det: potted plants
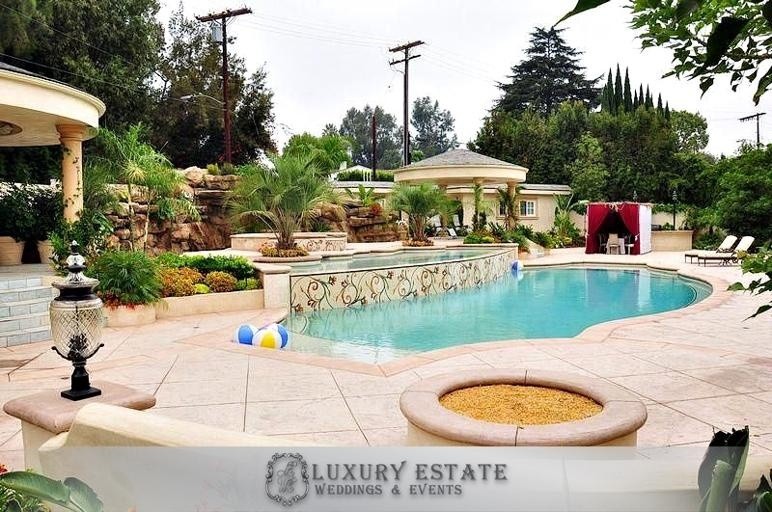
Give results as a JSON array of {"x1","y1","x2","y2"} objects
[{"x1": 0, "y1": 172, "x2": 71, "y2": 267}]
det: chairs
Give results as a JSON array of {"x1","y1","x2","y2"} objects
[
  {"x1": 684, "y1": 234, "x2": 755, "y2": 267},
  {"x1": 598, "y1": 232, "x2": 634, "y2": 255},
  {"x1": 431, "y1": 215, "x2": 469, "y2": 240}
]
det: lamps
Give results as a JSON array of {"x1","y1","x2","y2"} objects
[{"x1": 49, "y1": 238, "x2": 107, "y2": 401}]
